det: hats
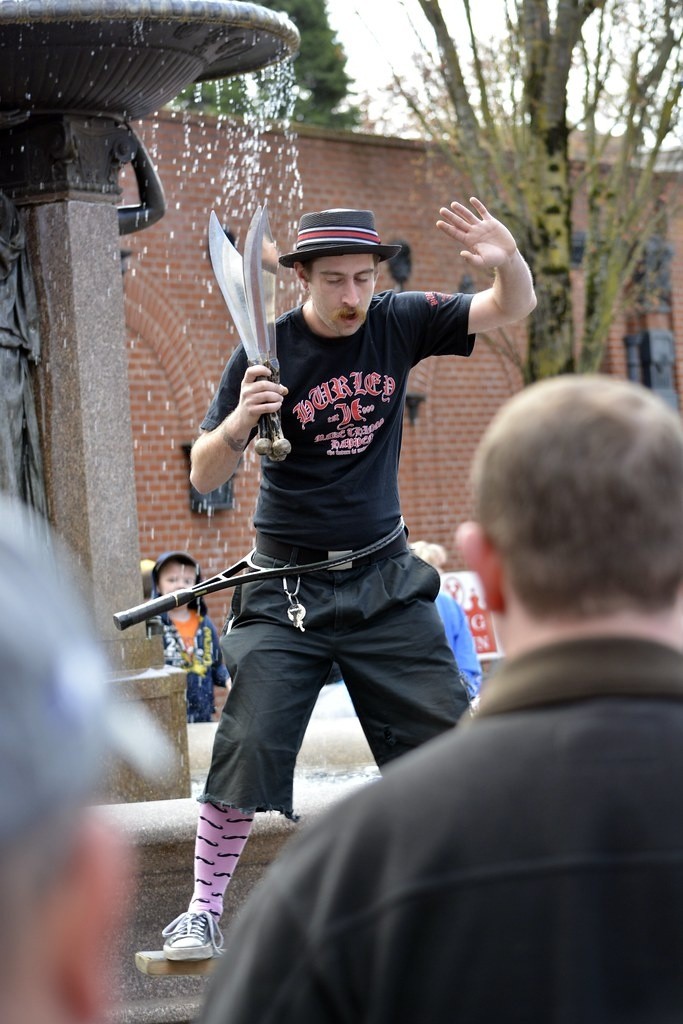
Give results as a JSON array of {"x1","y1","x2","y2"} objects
[{"x1": 278, "y1": 209, "x2": 403, "y2": 268}]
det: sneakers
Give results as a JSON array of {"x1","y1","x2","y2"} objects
[{"x1": 163, "y1": 911, "x2": 224, "y2": 961}]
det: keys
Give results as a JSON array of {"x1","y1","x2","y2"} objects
[{"x1": 285, "y1": 604, "x2": 306, "y2": 632}]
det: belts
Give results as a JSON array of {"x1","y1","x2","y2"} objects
[{"x1": 255, "y1": 529, "x2": 408, "y2": 569}]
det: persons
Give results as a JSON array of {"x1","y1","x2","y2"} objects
[
  {"x1": 194, "y1": 372, "x2": 682, "y2": 1024},
  {"x1": 146, "y1": 551, "x2": 232, "y2": 723},
  {"x1": 164, "y1": 195, "x2": 537, "y2": 962},
  {"x1": 0, "y1": 500, "x2": 141, "y2": 1024},
  {"x1": 409, "y1": 541, "x2": 481, "y2": 700}
]
